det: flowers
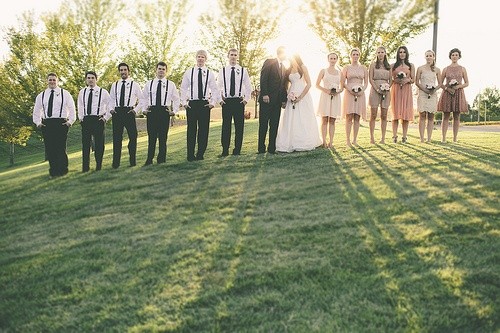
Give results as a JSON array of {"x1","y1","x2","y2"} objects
[
  {"x1": 426, "y1": 85, "x2": 435, "y2": 99},
  {"x1": 448, "y1": 79, "x2": 457, "y2": 96},
  {"x1": 287, "y1": 90, "x2": 297, "y2": 109},
  {"x1": 331, "y1": 87, "x2": 336, "y2": 100},
  {"x1": 396, "y1": 71, "x2": 407, "y2": 87},
  {"x1": 380, "y1": 83, "x2": 390, "y2": 99},
  {"x1": 352, "y1": 86, "x2": 362, "y2": 102}
]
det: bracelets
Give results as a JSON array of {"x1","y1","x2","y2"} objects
[
  {"x1": 377, "y1": 89, "x2": 379, "y2": 91},
  {"x1": 445, "y1": 87, "x2": 448, "y2": 91}
]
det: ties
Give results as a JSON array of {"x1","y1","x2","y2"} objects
[
  {"x1": 156, "y1": 80, "x2": 162, "y2": 112},
  {"x1": 230, "y1": 67, "x2": 236, "y2": 96},
  {"x1": 87, "y1": 89, "x2": 94, "y2": 116},
  {"x1": 120, "y1": 80, "x2": 126, "y2": 107},
  {"x1": 198, "y1": 67, "x2": 203, "y2": 100},
  {"x1": 279, "y1": 62, "x2": 283, "y2": 79},
  {"x1": 47, "y1": 90, "x2": 55, "y2": 117}
]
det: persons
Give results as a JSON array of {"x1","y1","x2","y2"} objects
[
  {"x1": 32, "y1": 73, "x2": 76, "y2": 180},
  {"x1": 341, "y1": 49, "x2": 368, "y2": 147},
  {"x1": 416, "y1": 50, "x2": 441, "y2": 144},
  {"x1": 218, "y1": 49, "x2": 250, "y2": 158},
  {"x1": 255, "y1": 46, "x2": 288, "y2": 154},
  {"x1": 181, "y1": 50, "x2": 217, "y2": 161},
  {"x1": 275, "y1": 51, "x2": 321, "y2": 153},
  {"x1": 142, "y1": 62, "x2": 180, "y2": 165},
  {"x1": 77, "y1": 71, "x2": 110, "y2": 172},
  {"x1": 437, "y1": 48, "x2": 469, "y2": 144},
  {"x1": 368, "y1": 47, "x2": 391, "y2": 145},
  {"x1": 390, "y1": 46, "x2": 415, "y2": 143},
  {"x1": 315, "y1": 53, "x2": 341, "y2": 148},
  {"x1": 109, "y1": 63, "x2": 143, "y2": 168}
]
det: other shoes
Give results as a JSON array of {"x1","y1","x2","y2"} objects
[
  {"x1": 323, "y1": 143, "x2": 332, "y2": 148},
  {"x1": 441, "y1": 138, "x2": 447, "y2": 144},
  {"x1": 392, "y1": 135, "x2": 407, "y2": 144},
  {"x1": 423, "y1": 138, "x2": 432, "y2": 144}
]
